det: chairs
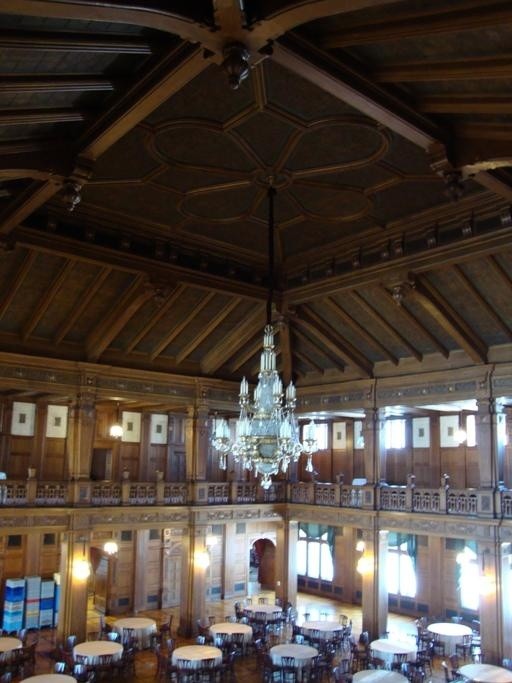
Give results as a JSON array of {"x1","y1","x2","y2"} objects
[
  {"x1": 155, "y1": 612, "x2": 512, "y2": 683},
  {"x1": 99, "y1": 615, "x2": 173, "y2": 653},
  {"x1": 0, "y1": 627, "x2": 138, "y2": 683},
  {"x1": 234, "y1": 597, "x2": 292, "y2": 631}
]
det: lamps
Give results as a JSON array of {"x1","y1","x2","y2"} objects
[
  {"x1": 59, "y1": 175, "x2": 86, "y2": 212},
  {"x1": 219, "y1": 37, "x2": 254, "y2": 92},
  {"x1": 208, "y1": 181, "x2": 318, "y2": 490}
]
